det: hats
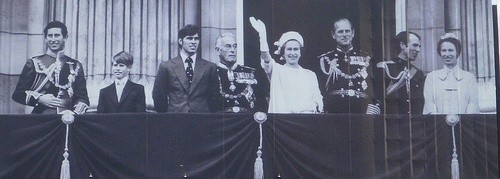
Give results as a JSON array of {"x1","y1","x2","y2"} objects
[
  {"x1": 441, "y1": 33, "x2": 458, "y2": 39},
  {"x1": 274, "y1": 31, "x2": 304, "y2": 54}
]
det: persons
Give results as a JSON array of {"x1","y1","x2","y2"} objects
[
  {"x1": 209, "y1": 32, "x2": 268, "y2": 114},
  {"x1": 152, "y1": 24, "x2": 220, "y2": 113},
  {"x1": 377, "y1": 31, "x2": 425, "y2": 114},
  {"x1": 12, "y1": 20, "x2": 90, "y2": 115},
  {"x1": 97, "y1": 51, "x2": 145, "y2": 113},
  {"x1": 422, "y1": 37, "x2": 481, "y2": 114},
  {"x1": 249, "y1": 16, "x2": 324, "y2": 114},
  {"x1": 314, "y1": 17, "x2": 382, "y2": 114}
]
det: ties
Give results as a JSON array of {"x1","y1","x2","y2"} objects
[
  {"x1": 117, "y1": 81, "x2": 124, "y2": 103},
  {"x1": 185, "y1": 57, "x2": 193, "y2": 89}
]
